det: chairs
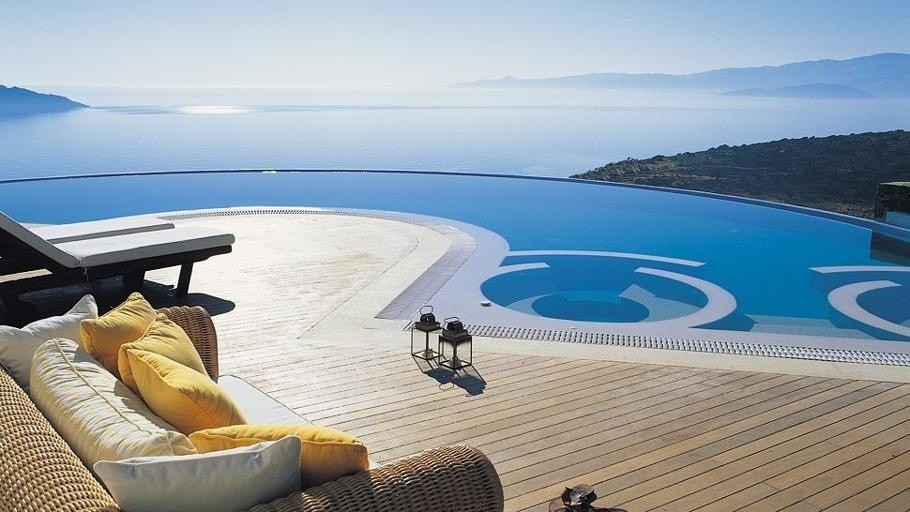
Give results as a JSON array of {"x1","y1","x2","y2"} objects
[
  {"x1": 28, "y1": 214, "x2": 176, "y2": 241},
  {"x1": 1, "y1": 210, "x2": 235, "y2": 306}
]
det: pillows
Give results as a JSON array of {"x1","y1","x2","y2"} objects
[
  {"x1": 118, "y1": 312, "x2": 209, "y2": 399},
  {"x1": 1, "y1": 292, "x2": 96, "y2": 389},
  {"x1": 22, "y1": 334, "x2": 197, "y2": 471},
  {"x1": 81, "y1": 291, "x2": 158, "y2": 378},
  {"x1": 191, "y1": 421, "x2": 369, "y2": 489},
  {"x1": 124, "y1": 347, "x2": 245, "y2": 438},
  {"x1": 91, "y1": 434, "x2": 303, "y2": 512}
]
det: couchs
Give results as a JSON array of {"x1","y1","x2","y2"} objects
[{"x1": 0, "y1": 292, "x2": 505, "y2": 511}]
